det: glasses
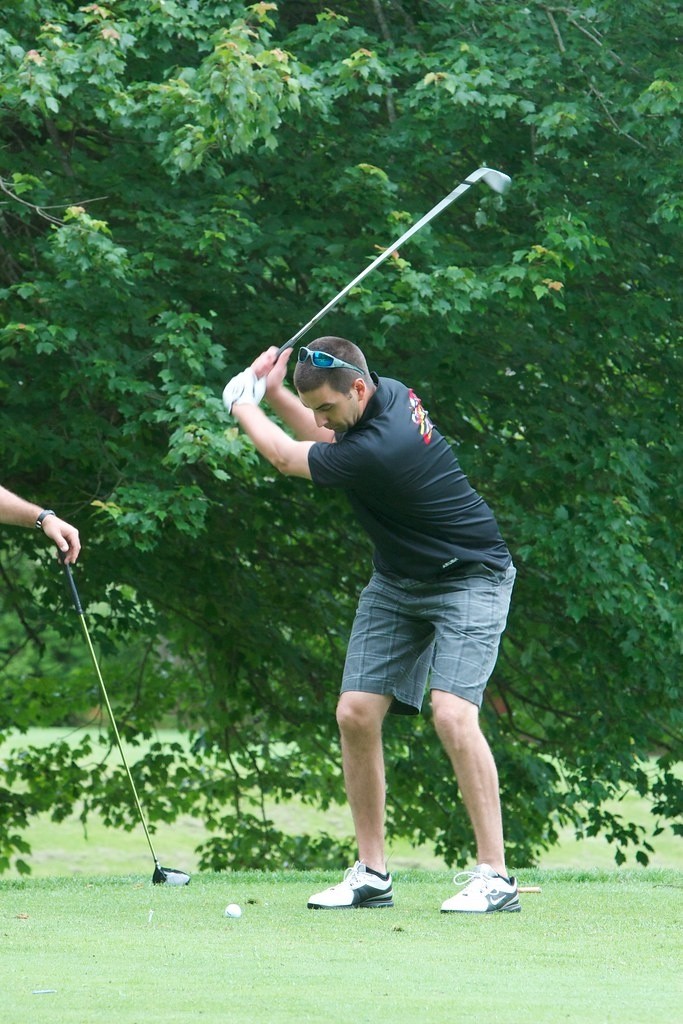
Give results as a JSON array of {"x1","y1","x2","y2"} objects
[{"x1": 298, "y1": 347, "x2": 365, "y2": 376}]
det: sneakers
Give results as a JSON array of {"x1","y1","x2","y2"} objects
[
  {"x1": 307, "y1": 858, "x2": 394, "y2": 909},
  {"x1": 441, "y1": 864, "x2": 521, "y2": 914}
]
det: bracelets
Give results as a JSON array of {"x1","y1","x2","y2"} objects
[{"x1": 35, "y1": 509, "x2": 55, "y2": 532}]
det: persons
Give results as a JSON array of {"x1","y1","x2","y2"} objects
[
  {"x1": 0, "y1": 486, "x2": 81, "y2": 564},
  {"x1": 223, "y1": 336, "x2": 521, "y2": 916}
]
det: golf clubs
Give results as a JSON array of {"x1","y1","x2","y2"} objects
[
  {"x1": 62, "y1": 551, "x2": 189, "y2": 885},
  {"x1": 274, "y1": 167, "x2": 511, "y2": 358}
]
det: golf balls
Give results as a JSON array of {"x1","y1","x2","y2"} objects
[{"x1": 225, "y1": 904, "x2": 242, "y2": 918}]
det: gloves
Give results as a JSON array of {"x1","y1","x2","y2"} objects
[{"x1": 222, "y1": 367, "x2": 265, "y2": 415}]
